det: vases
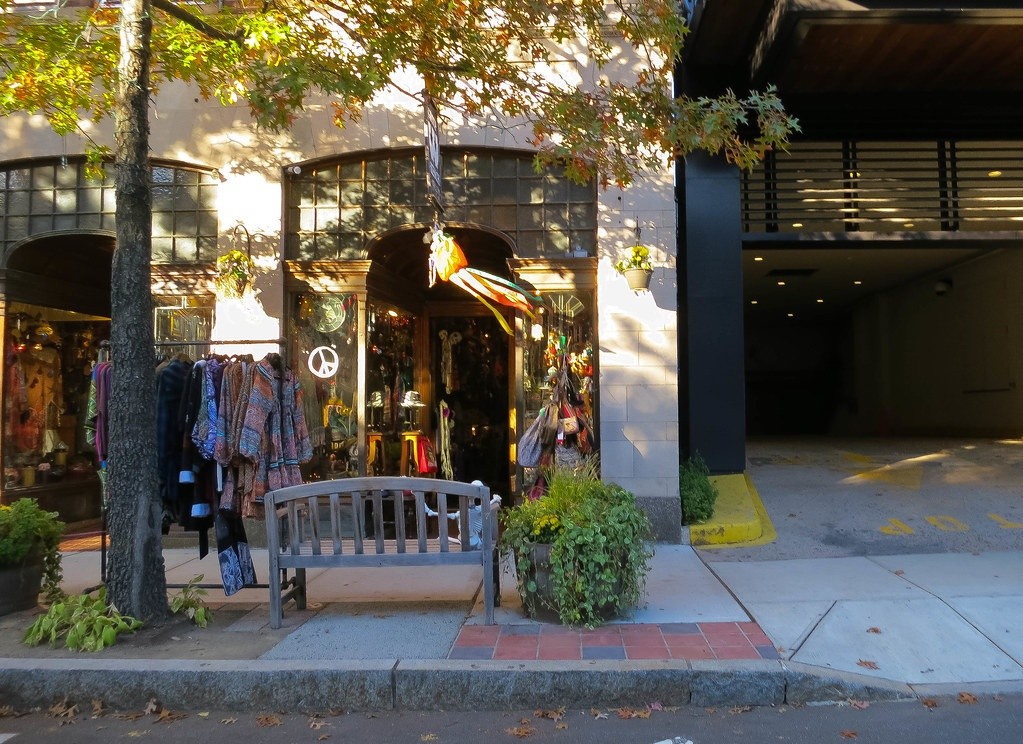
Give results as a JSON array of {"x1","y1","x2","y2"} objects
[
  {"x1": 513, "y1": 541, "x2": 625, "y2": 626},
  {"x1": 0, "y1": 555, "x2": 43, "y2": 615}
]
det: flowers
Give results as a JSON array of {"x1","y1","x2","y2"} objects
[
  {"x1": 0, "y1": 496, "x2": 66, "y2": 605},
  {"x1": 493, "y1": 450, "x2": 659, "y2": 632}
]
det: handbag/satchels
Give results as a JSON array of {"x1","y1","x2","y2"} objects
[
  {"x1": 416, "y1": 434, "x2": 439, "y2": 476},
  {"x1": 517, "y1": 365, "x2": 594, "y2": 468}
]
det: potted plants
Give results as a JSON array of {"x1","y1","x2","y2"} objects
[
  {"x1": 615, "y1": 244, "x2": 655, "y2": 292},
  {"x1": 214, "y1": 249, "x2": 253, "y2": 298}
]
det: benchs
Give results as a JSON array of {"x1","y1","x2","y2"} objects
[{"x1": 265, "y1": 475, "x2": 502, "y2": 629}]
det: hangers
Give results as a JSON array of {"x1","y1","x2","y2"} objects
[{"x1": 155, "y1": 341, "x2": 289, "y2": 371}]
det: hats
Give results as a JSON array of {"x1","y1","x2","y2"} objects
[
  {"x1": 367, "y1": 391, "x2": 384, "y2": 408},
  {"x1": 400, "y1": 390, "x2": 427, "y2": 408}
]
